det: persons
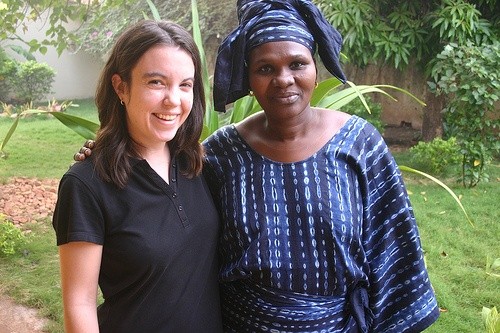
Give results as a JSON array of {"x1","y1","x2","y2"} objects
[
  {"x1": 72, "y1": 0, "x2": 440, "y2": 333},
  {"x1": 52, "y1": 18, "x2": 226, "y2": 333}
]
